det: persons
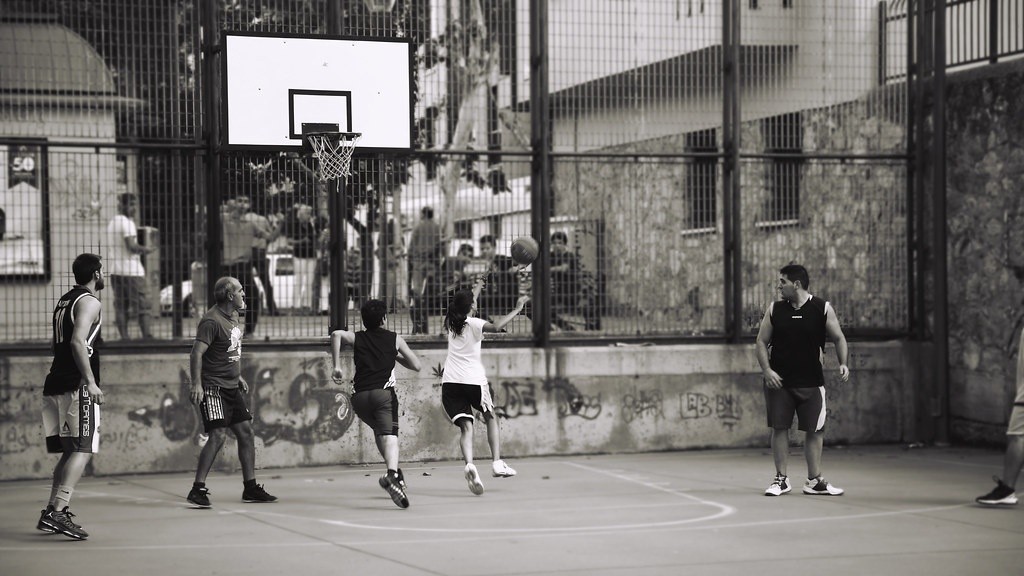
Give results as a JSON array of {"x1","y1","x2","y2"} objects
[
  {"x1": 976, "y1": 327, "x2": 1024, "y2": 505},
  {"x1": 35, "y1": 193, "x2": 580, "y2": 541},
  {"x1": 755, "y1": 264, "x2": 849, "y2": 497}
]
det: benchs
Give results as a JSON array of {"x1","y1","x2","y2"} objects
[{"x1": 423, "y1": 271, "x2": 593, "y2": 333}]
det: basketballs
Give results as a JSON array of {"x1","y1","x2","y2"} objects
[{"x1": 510, "y1": 236, "x2": 540, "y2": 264}]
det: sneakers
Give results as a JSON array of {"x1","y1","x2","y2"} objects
[
  {"x1": 464, "y1": 463, "x2": 484, "y2": 495},
  {"x1": 379, "y1": 470, "x2": 408, "y2": 508},
  {"x1": 802, "y1": 472, "x2": 843, "y2": 495},
  {"x1": 242, "y1": 484, "x2": 279, "y2": 503},
  {"x1": 41, "y1": 505, "x2": 89, "y2": 539},
  {"x1": 37, "y1": 510, "x2": 63, "y2": 533},
  {"x1": 492, "y1": 460, "x2": 517, "y2": 477},
  {"x1": 976, "y1": 476, "x2": 1018, "y2": 504},
  {"x1": 764, "y1": 471, "x2": 792, "y2": 495},
  {"x1": 186, "y1": 486, "x2": 212, "y2": 507}
]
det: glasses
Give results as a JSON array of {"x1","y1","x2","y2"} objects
[{"x1": 462, "y1": 251, "x2": 472, "y2": 258}]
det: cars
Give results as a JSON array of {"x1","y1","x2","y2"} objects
[{"x1": 156, "y1": 252, "x2": 355, "y2": 320}]
